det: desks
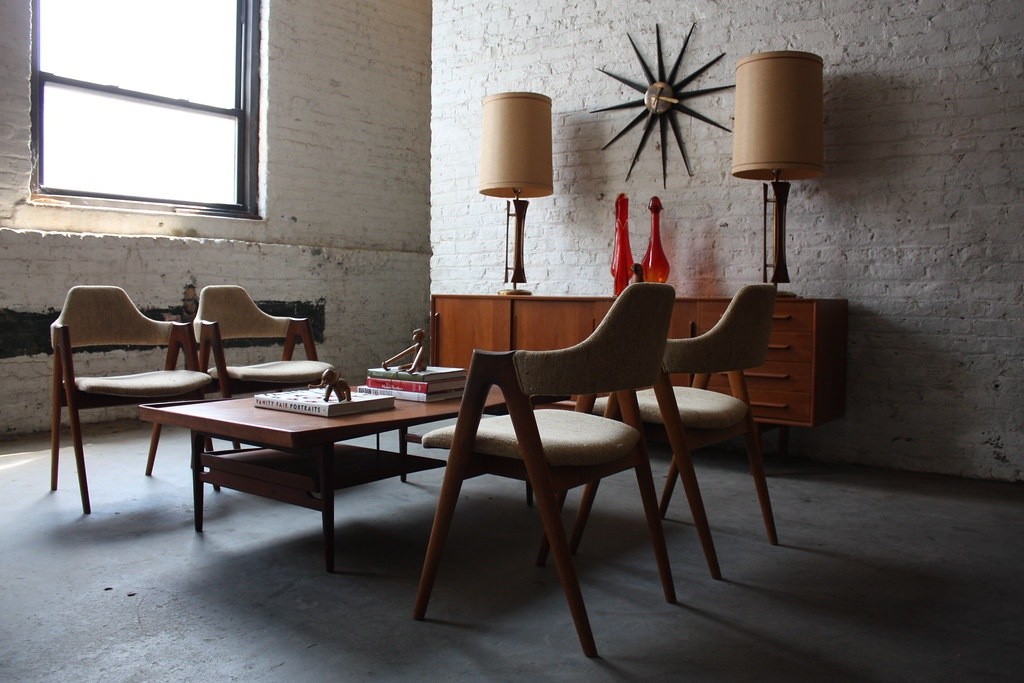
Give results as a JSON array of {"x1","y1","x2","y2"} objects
[{"x1": 138, "y1": 385, "x2": 572, "y2": 572}]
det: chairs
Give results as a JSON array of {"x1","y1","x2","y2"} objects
[
  {"x1": 49, "y1": 284, "x2": 221, "y2": 514},
  {"x1": 193, "y1": 283, "x2": 334, "y2": 450},
  {"x1": 567, "y1": 282, "x2": 779, "y2": 578},
  {"x1": 413, "y1": 279, "x2": 676, "y2": 658}
]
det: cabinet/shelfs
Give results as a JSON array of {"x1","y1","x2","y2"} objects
[{"x1": 431, "y1": 294, "x2": 849, "y2": 470}]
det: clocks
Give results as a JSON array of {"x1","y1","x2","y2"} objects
[{"x1": 590, "y1": 23, "x2": 737, "y2": 189}]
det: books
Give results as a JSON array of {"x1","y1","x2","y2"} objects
[
  {"x1": 253, "y1": 388, "x2": 396, "y2": 417},
  {"x1": 357, "y1": 365, "x2": 468, "y2": 403}
]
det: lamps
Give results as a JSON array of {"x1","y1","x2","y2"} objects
[
  {"x1": 731, "y1": 50, "x2": 824, "y2": 299},
  {"x1": 479, "y1": 92, "x2": 553, "y2": 295}
]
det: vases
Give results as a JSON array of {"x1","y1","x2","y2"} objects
[
  {"x1": 641, "y1": 196, "x2": 670, "y2": 283},
  {"x1": 609, "y1": 193, "x2": 634, "y2": 297}
]
